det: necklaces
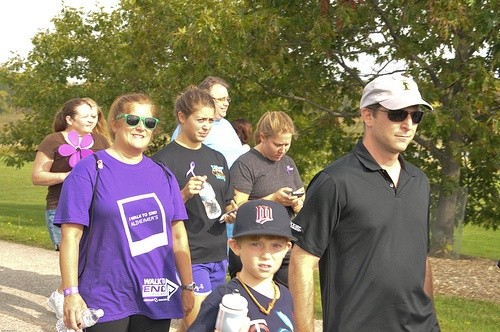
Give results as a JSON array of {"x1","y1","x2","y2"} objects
[{"x1": 236, "y1": 273, "x2": 279, "y2": 315}]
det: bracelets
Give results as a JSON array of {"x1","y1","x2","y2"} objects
[{"x1": 62, "y1": 286, "x2": 78, "y2": 296}]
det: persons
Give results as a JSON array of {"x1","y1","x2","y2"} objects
[
  {"x1": 31, "y1": 99, "x2": 110, "y2": 320},
  {"x1": 229, "y1": 111, "x2": 306, "y2": 287},
  {"x1": 186, "y1": 199, "x2": 296, "y2": 332},
  {"x1": 147, "y1": 85, "x2": 239, "y2": 332},
  {"x1": 289, "y1": 73, "x2": 440, "y2": 332},
  {"x1": 172, "y1": 75, "x2": 243, "y2": 239},
  {"x1": 82, "y1": 97, "x2": 112, "y2": 153},
  {"x1": 231, "y1": 119, "x2": 252, "y2": 153},
  {"x1": 53, "y1": 94, "x2": 195, "y2": 332}
]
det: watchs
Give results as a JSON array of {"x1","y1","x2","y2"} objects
[{"x1": 183, "y1": 282, "x2": 196, "y2": 291}]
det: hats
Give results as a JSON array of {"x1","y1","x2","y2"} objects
[
  {"x1": 232, "y1": 199, "x2": 298, "y2": 241},
  {"x1": 360, "y1": 75, "x2": 433, "y2": 112}
]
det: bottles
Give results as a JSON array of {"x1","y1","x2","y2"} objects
[
  {"x1": 56, "y1": 308, "x2": 104, "y2": 332},
  {"x1": 215, "y1": 289, "x2": 250, "y2": 332},
  {"x1": 199, "y1": 182, "x2": 221, "y2": 219}
]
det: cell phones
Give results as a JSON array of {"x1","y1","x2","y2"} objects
[
  {"x1": 219, "y1": 208, "x2": 238, "y2": 223},
  {"x1": 292, "y1": 191, "x2": 304, "y2": 198}
]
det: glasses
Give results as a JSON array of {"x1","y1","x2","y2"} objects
[
  {"x1": 365, "y1": 107, "x2": 424, "y2": 124},
  {"x1": 115, "y1": 113, "x2": 159, "y2": 130},
  {"x1": 212, "y1": 97, "x2": 231, "y2": 104}
]
